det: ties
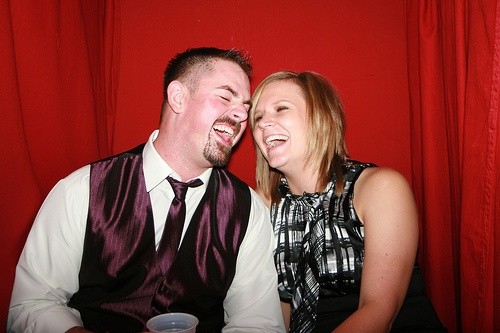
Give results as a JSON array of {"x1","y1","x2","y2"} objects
[{"x1": 158, "y1": 176, "x2": 204, "y2": 276}]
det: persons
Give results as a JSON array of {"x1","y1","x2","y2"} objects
[
  {"x1": 246, "y1": 70, "x2": 446, "y2": 333},
  {"x1": 3, "y1": 45, "x2": 287, "y2": 333}
]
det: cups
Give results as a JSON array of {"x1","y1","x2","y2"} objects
[{"x1": 146, "y1": 313, "x2": 199, "y2": 333}]
